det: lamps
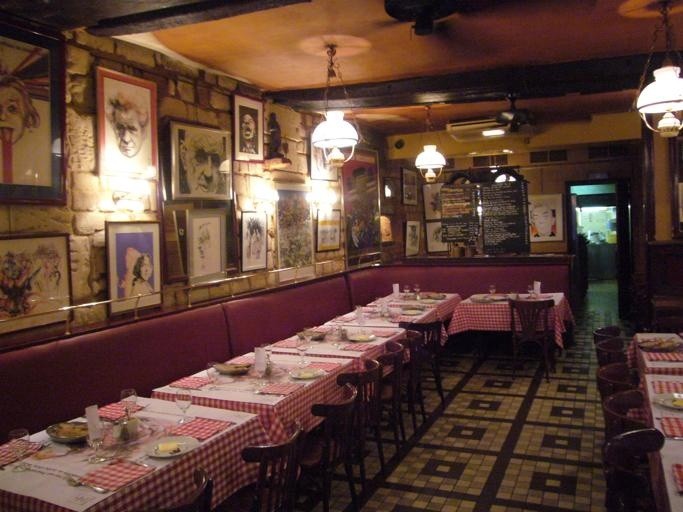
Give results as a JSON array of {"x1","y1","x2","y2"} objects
[
  {"x1": 414, "y1": 101, "x2": 448, "y2": 184},
  {"x1": 308, "y1": 37, "x2": 363, "y2": 170},
  {"x1": 635, "y1": 1, "x2": 682, "y2": 139}
]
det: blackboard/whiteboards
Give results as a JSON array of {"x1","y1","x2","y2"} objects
[
  {"x1": 481, "y1": 180, "x2": 530, "y2": 254},
  {"x1": 439, "y1": 184, "x2": 479, "y2": 242}
]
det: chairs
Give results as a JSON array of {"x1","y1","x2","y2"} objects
[
  {"x1": 297, "y1": 382, "x2": 368, "y2": 511},
  {"x1": 508, "y1": 298, "x2": 556, "y2": 383},
  {"x1": 240, "y1": 419, "x2": 305, "y2": 511},
  {"x1": 396, "y1": 330, "x2": 428, "y2": 437},
  {"x1": 413, "y1": 321, "x2": 443, "y2": 403},
  {"x1": 596, "y1": 361, "x2": 639, "y2": 401},
  {"x1": 594, "y1": 326, "x2": 624, "y2": 345},
  {"x1": 602, "y1": 390, "x2": 647, "y2": 447},
  {"x1": 596, "y1": 338, "x2": 626, "y2": 367},
  {"x1": 601, "y1": 428, "x2": 665, "y2": 511}
]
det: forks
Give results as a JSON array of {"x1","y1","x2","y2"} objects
[{"x1": 64, "y1": 475, "x2": 110, "y2": 494}]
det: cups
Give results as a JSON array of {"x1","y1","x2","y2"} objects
[
  {"x1": 356, "y1": 297, "x2": 389, "y2": 322},
  {"x1": 206, "y1": 361, "x2": 217, "y2": 385}
]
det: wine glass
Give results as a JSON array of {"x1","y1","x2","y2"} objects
[
  {"x1": 176, "y1": 388, "x2": 193, "y2": 425},
  {"x1": 329, "y1": 313, "x2": 347, "y2": 348},
  {"x1": 86, "y1": 420, "x2": 109, "y2": 464},
  {"x1": 489, "y1": 285, "x2": 552, "y2": 301},
  {"x1": 121, "y1": 389, "x2": 137, "y2": 420},
  {"x1": 9, "y1": 429, "x2": 30, "y2": 472},
  {"x1": 296, "y1": 337, "x2": 312, "y2": 368}
]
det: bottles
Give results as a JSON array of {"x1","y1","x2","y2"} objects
[
  {"x1": 113, "y1": 418, "x2": 137, "y2": 444},
  {"x1": 264, "y1": 348, "x2": 273, "y2": 376}
]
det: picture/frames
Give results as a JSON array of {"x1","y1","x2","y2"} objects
[
  {"x1": 104, "y1": 220, "x2": 163, "y2": 317},
  {"x1": 398, "y1": 167, "x2": 564, "y2": 258},
  {"x1": 1, "y1": 24, "x2": 67, "y2": 206},
  {"x1": 241, "y1": 211, "x2": 267, "y2": 272},
  {"x1": 233, "y1": 94, "x2": 265, "y2": 163},
  {"x1": 1, "y1": 233, "x2": 75, "y2": 335},
  {"x1": 96, "y1": 66, "x2": 161, "y2": 213},
  {"x1": 185, "y1": 210, "x2": 228, "y2": 285},
  {"x1": 170, "y1": 121, "x2": 233, "y2": 201}
]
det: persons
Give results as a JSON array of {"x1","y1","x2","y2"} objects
[
  {"x1": 131, "y1": 254, "x2": 152, "y2": 296},
  {"x1": 105, "y1": 93, "x2": 149, "y2": 158},
  {"x1": 241, "y1": 113, "x2": 257, "y2": 154},
  {"x1": 532, "y1": 201, "x2": 555, "y2": 236},
  {"x1": 182, "y1": 135, "x2": 224, "y2": 195},
  {"x1": 0, "y1": 71, "x2": 41, "y2": 146}
]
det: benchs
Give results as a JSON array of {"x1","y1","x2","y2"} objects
[
  {"x1": 354, "y1": 261, "x2": 576, "y2": 346},
  {"x1": 221, "y1": 273, "x2": 354, "y2": 357},
  {"x1": 0, "y1": 300, "x2": 236, "y2": 446}
]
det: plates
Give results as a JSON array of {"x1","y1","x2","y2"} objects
[
  {"x1": 296, "y1": 331, "x2": 326, "y2": 340},
  {"x1": 213, "y1": 363, "x2": 252, "y2": 375},
  {"x1": 402, "y1": 310, "x2": 422, "y2": 315},
  {"x1": 392, "y1": 283, "x2": 445, "y2": 306},
  {"x1": 145, "y1": 436, "x2": 200, "y2": 458},
  {"x1": 471, "y1": 295, "x2": 494, "y2": 303},
  {"x1": 346, "y1": 334, "x2": 376, "y2": 342},
  {"x1": 290, "y1": 368, "x2": 326, "y2": 378},
  {"x1": 46, "y1": 421, "x2": 89, "y2": 444},
  {"x1": 640, "y1": 337, "x2": 683, "y2": 410}
]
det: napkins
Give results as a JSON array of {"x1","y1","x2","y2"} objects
[
  {"x1": 82, "y1": 460, "x2": 156, "y2": 492},
  {"x1": 1, "y1": 440, "x2": 46, "y2": 466},
  {"x1": 99, "y1": 401, "x2": 145, "y2": 422}
]
